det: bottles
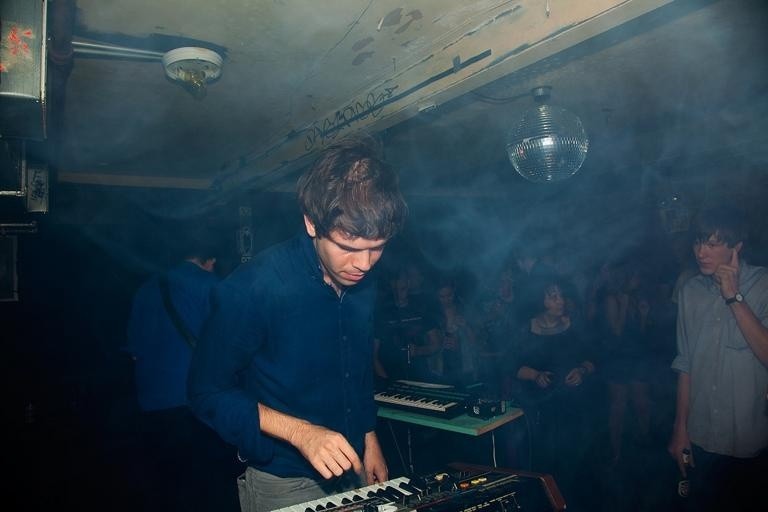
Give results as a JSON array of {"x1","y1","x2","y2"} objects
[{"x1": 675, "y1": 447, "x2": 695, "y2": 505}]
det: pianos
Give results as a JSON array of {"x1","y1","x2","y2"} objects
[
  {"x1": 373, "y1": 379, "x2": 482, "y2": 419},
  {"x1": 267, "y1": 462, "x2": 567, "y2": 512}
]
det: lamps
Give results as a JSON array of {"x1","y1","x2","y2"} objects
[{"x1": 502, "y1": 85, "x2": 588, "y2": 184}]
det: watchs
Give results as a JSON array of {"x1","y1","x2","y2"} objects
[{"x1": 725, "y1": 293, "x2": 745, "y2": 303}]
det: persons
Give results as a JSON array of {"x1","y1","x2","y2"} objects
[
  {"x1": 607, "y1": 263, "x2": 653, "y2": 446},
  {"x1": 513, "y1": 281, "x2": 603, "y2": 469},
  {"x1": 434, "y1": 278, "x2": 479, "y2": 374},
  {"x1": 186, "y1": 135, "x2": 409, "y2": 512},
  {"x1": 122, "y1": 233, "x2": 220, "y2": 511},
  {"x1": 372, "y1": 275, "x2": 439, "y2": 381},
  {"x1": 670, "y1": 206, "x2": 768, "y2": 477}
]
